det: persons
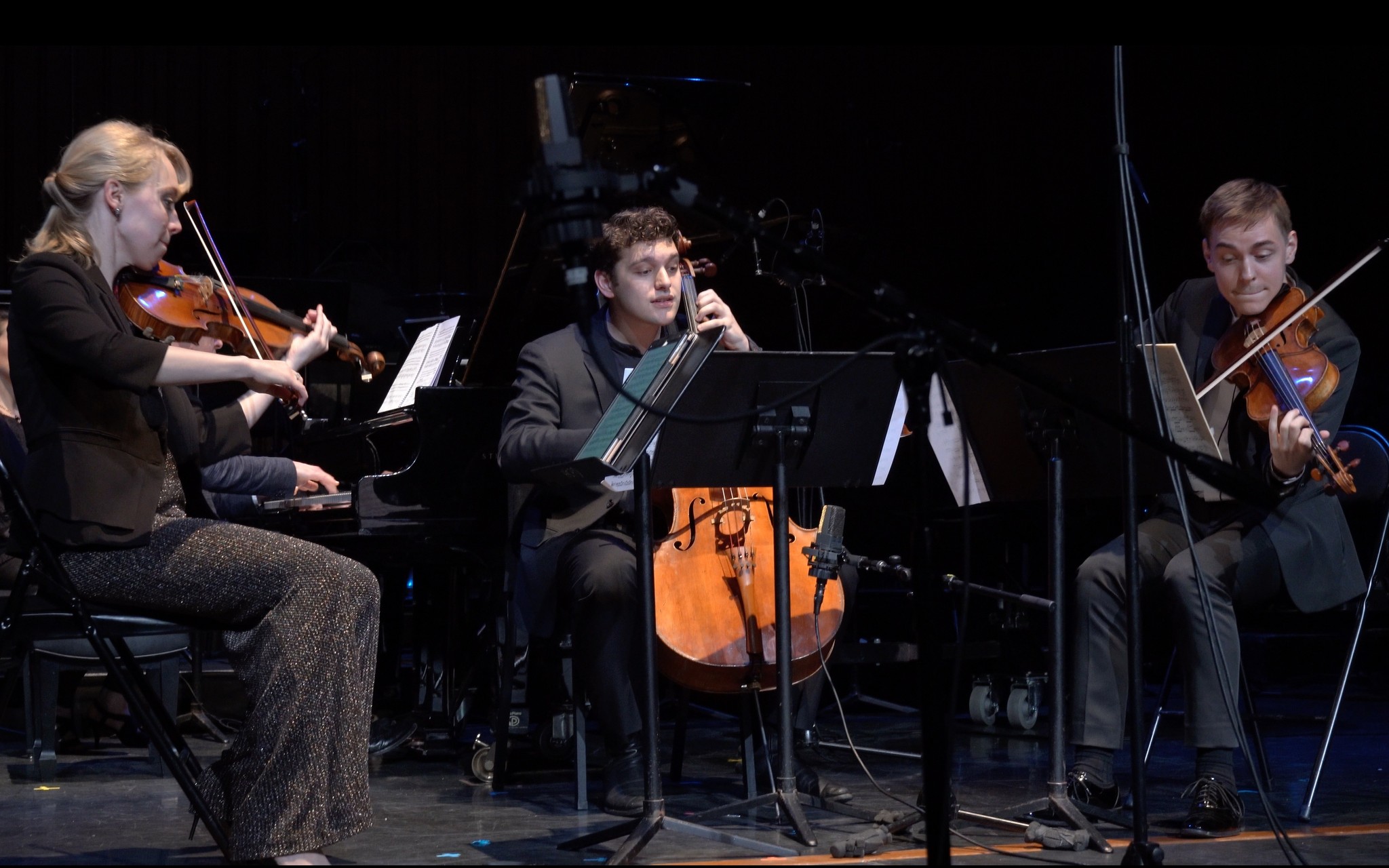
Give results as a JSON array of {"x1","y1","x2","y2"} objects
[
  {"x1": 1014, "y1": 178, "x2": 1368, "y2": 841},
  {"x1": 494, "y1": 204, "x2": 852, "y2": 817},
  {"x1": 1, "y1": 120, "x2": 383, "y2": 866}
]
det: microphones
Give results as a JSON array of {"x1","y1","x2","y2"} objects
[
  {"x1": 811, "y1": 214, "x2": 822, "y2": 254},
  {"x1": 534, "y1": 73, "x2": 580, "y2": 202},
  {"x1": 721, "y1": 202, "x2": 774, "y2": 259},
  {"x1": 812, "y1": 504, "x2": 845, "y2": 615}
]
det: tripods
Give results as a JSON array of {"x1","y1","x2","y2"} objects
[
  {"x1": 178, "y1": 631, "x2": 232, "y2": 744},
  {"x1": 529, "y1": 326, "x2": 1218, "y2": 868}
]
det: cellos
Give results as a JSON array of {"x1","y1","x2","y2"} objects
[{"x1": 647, "y1": 224, "x2": 849, "y2": 819}]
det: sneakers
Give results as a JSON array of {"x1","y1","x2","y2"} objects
[
  {"x1": 1014, "y1": 769, "x2": 1129, "y2": 828},
  {"x1": 1179, "y1": 776, "x2": 1245, "y2": 840}
]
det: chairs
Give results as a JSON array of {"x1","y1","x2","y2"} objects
[
  {"x1": 485, "y1": 452, "x2": 762, "y2": 812},
  {"x1": 1122, "y1": 424, "x2": 1389, "y2": 827},
  {"x1": 0, "y1": 413, "x2": 247, "y2": 868}
]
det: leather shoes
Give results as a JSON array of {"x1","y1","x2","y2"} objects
[
  {"x1": 752, "y1": 753, "x2": 855, "y2": 806},
  {"x1": 599, "y1": 733, "x2": 649, "y2": 815}
]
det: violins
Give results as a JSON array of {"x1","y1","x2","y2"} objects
[
  {"x1": 114, "y1": 260, "x2": 385, "y2": 380},
  {"x1": 1212, "y1": 283, "x2": 1361, "y2": 498}
]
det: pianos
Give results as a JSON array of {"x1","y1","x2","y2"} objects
[{"x1": 253, "y1": 388, "x2": 521, "y2": 778}]
